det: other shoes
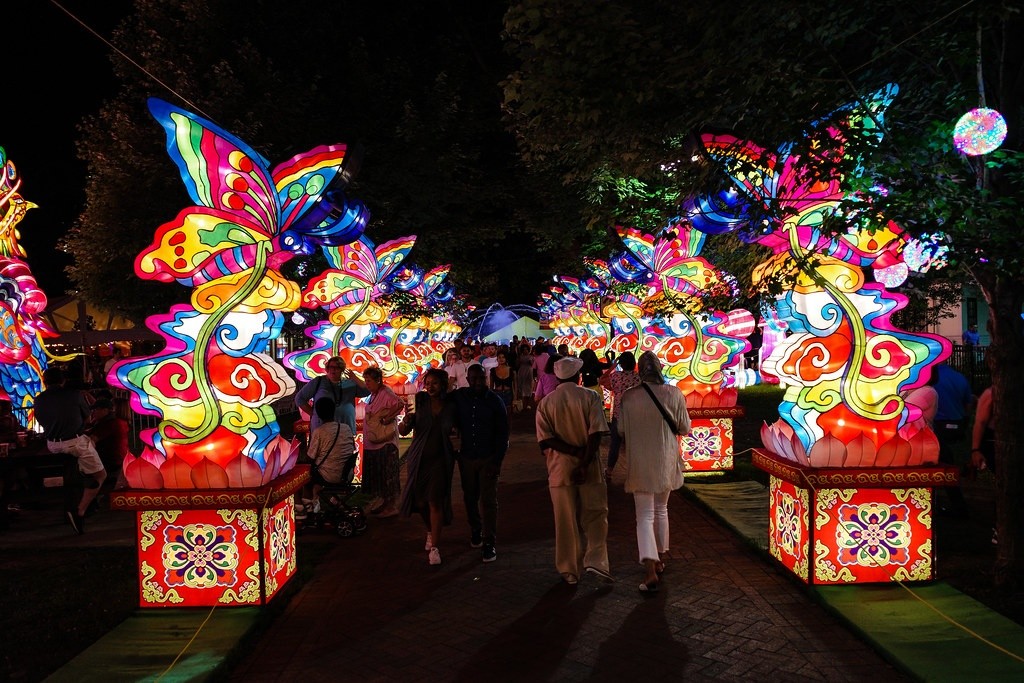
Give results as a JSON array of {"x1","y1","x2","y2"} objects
[
  {"x1": 371, "y1": 502, "x2": 386, "y2": 513},
  {"x1": 561, "y1": 572, "x2": 578, "y2": 584},
  {"x1": 67, "y1": 503, "x2": 85, "y2": 534},
  {"x1": 585, "y1": 566, "x2": 617, "y2": 583},
  {"x1": 294, "y1": 507, "x2": 307, "y2": 520},
  {"x1": 376, "y1": 508, "x2": 399, "y2": 519},
  {"x1": 425, "y1": 532, "x2": 436, "y2": 551},
  {"x1": 429, "y1": 547, "x2": 441, "y2": 564},
  {"x1": 302, "y1": 502, "x2": 316, "y2": 514}
]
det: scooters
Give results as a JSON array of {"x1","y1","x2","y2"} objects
[{"x1": 294, "y1": 481, "x2": 372, "y2": 539}]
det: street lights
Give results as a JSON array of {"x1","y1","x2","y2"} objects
[{"x1": 951, "y1": 107, "x2": 1007, "y2": 315}]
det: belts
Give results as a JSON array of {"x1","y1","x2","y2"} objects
[{"x1": 48, "y1": 433, "x2": 83, "y2": 442}]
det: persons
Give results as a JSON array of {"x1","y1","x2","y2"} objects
[
  {"x1": 295, "y1": 332, "x2": 641, "y2": 565},
  {"x1": 619, "y1": 350, "x2": 688, "y2": 589},
  {"x1": 536, "y1": 356, "x2": 614, "y2": 584},
  {"x1": 0, "y1": 367, "x2": 130, "y2": 536},
  {"x1": 929, "y1": 318, "x2": 995, "y2": 541}
]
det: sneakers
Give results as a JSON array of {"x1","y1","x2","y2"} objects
[
  {"x1": 483, "y1": 538, "x2": 497, "y2": 562},
  {"x1": 470, "y1": 528, "x2": 482, "y2": 547}
]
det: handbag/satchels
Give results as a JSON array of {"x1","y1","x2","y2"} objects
[
  {"x1": 367, "y1": 412, "x2": 397, "y2": 444},
  {"x1": 301, "y1": 461, "x2": 318, "y2": 487}
]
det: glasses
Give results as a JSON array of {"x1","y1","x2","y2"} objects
[{"x1": 329, "y1": 366, "x2": 341, "y2": 370}]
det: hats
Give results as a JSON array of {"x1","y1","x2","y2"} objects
[
  {"x1": 90, "y1": 399, "x2": 112, "y2": 409},
  {"x1": 554, "y1": 356, "x2": 584, "y2": 379}
]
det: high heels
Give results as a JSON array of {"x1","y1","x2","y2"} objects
[
  {"x1": 656, "y1": 562, "x2": 665, "y2": 574},
  {"x1": 639, "y1": 573, "x2": 660, "y2": 591}
]
